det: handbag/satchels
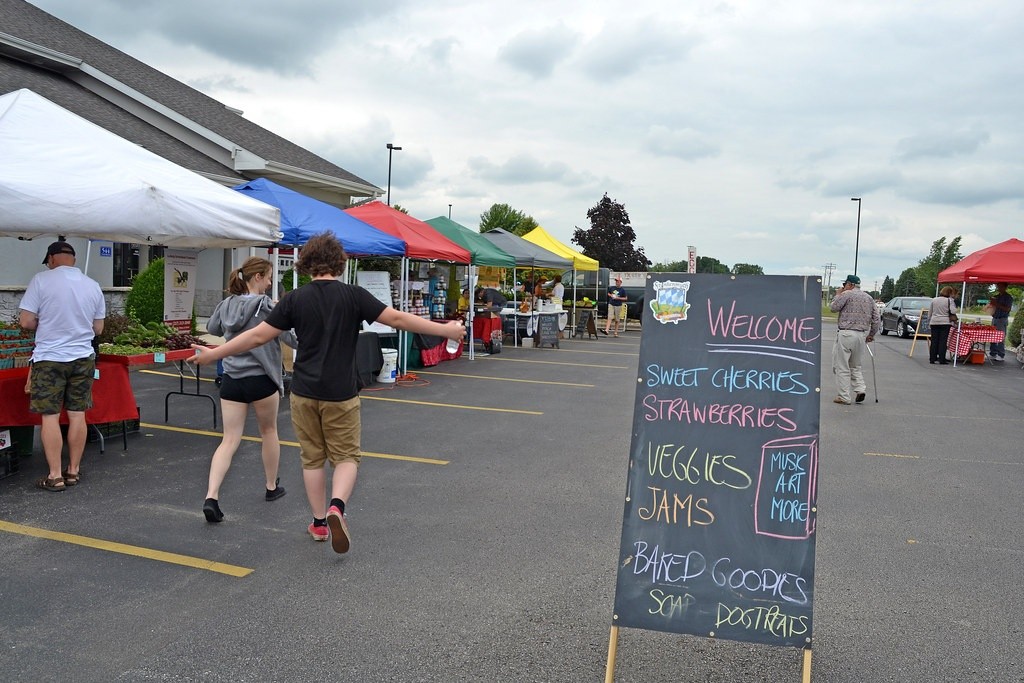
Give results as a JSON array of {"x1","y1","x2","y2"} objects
[
  {"x1": 982, "y1": 297, "x2": 997, "y2": 314},
  {"x1": 948, "y1": 298, "x2": 957, "y2": 322}
]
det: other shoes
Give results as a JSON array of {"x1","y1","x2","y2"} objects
[
  {"x1": 600, "y1": 329, "x2": 609, "y2": 335},
  {"x1": 614, "y1": 333, "x2": 618, "y2": 337},
  {"x1": 856, "y1": 393, "x2": 865, "y2": 402},
  {"x1": 930, "y1": 358, "x2": 951, "y2": 364},
  {"x1": 834, "y1": 397, "x2": 851, "y2": 405}
]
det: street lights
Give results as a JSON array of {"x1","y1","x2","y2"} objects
[
  {"x1": 387, "y1": 144, "x2": 402, "y2": 206},
  {"x1": 851, "y1": 198, "x2": 861, "y2": 276}
]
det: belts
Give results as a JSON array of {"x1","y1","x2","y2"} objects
[
  {"x1": 839, "y1": 328, "x2": 864, "y2": 332},
  {"x1": 993, "y1": 317, "x2": 1003, "y2": 319}
]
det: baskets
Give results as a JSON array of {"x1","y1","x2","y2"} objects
[{"x1": 0, "y1": 326, "x2": 35, "y2": 370}]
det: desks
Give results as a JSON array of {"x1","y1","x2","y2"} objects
[
  {"x1": 946, "y1": 326, "x2": 1004, "y2": 365},
  {"x1": 0, "y1": 362, "x2": 140, "y2": 454},
  {"x1": 420, "y1": 318, "x2": 463, "y2": 366},
  {"x1": 475, "y1": 317, "x2": 502, "y2": 353},
  {"x1": 97, "y1": 345, "x2": 219, "y2": 450},
  {"x1": 500, "y1": 307, "x2": 569, "y2": 345}
]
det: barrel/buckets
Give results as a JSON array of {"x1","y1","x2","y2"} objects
[{"x1": 377, "y1": 346, "x2": 398, "y2": 384}]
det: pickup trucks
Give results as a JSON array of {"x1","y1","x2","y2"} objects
[{"x1": 541, "y1": 268, "x2": 645, "y2": 319}]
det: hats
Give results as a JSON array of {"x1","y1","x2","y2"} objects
[
  {"x1": 475, "y1": 289, "x2": 481, "y2": 297},
  {"x1": 42, "y1": 242, "x2": 75, "y2": 264},
  {"x1": 841, "y1": 275, "x2": 860, "y2": 284},
  {"x1": 615, "y1": 277, "x2": 622, "y2": 283},
  {"x1": 541, "y1": 276, "x2": 549, "y2": 281}
]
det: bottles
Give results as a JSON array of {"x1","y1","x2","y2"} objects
[{"x1": 446, "y1": 322, "x2": 462, "y2": 354}]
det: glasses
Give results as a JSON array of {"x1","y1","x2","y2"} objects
[{"x1": 46, "y1": 262, "x2": 49, "y2": 266}]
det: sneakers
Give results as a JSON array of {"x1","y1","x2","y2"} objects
[
  {"x1": 203, "y1": 498, "x2": 224, "y2": 522},
  {"x1": 985, "y1": 355, "x2": 1005, "y2": 361},
  {"x1": 326, "y1": 505, "x2": 351, "y2": 553},
  {"x1": 307, "y1": 520, "x2": 328, "y2": 541},
  {"x1": 266, "y1": 478, "x2": 284, "y2": 501}
]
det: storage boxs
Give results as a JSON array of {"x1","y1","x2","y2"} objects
[
  {"x1": 970, "y1": 350, "x2": 985, "y2": 365},
  {"x1": 85, "y1": 406, "x2": 140, "y2": 444},
  {"x1": 0, "y1": 424, "x2": 20, "y2": 479}
]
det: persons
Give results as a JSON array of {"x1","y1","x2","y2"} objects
[
  {"x1": 19, "y1": 242, "x2": 108, "y2": 490},
  {"x1": 600, "y1": 278, "x2": 627, "y2": 338},
  {"x1": 186, "y1": 231, "x2": 467, "y2": 554},
  {"x1": 535, "y1": 276, "x2": 548, "y2": 294},
  {"x1": 829, "y1": 274, "x2": 880, "y2": 405},
  {"x1": 203, "y1": 256, "x2": 298, "y2": 523},
  {"x1": 985, "y1": 283, "x2": 1014, "y2": 360},
  {"x1": 546, "y1": 275, "x2": 564, "y2": 339},
  {"x1": 522, "y1": 273, "x2": 536, "y2": 293},
  {"x1": 458, "y1": 289, "x2": 469, "y2": 313},
  {"x1": 476, "y1": 287, "x2": 507, "y2": 307},
  {"x1": 929, "y1": 286, "x2": 957, "y2": 365}
]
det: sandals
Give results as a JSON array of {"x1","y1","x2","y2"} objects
[
  {"x1": 36, "y1": 472, "x2": 66, "y2": 492},
  {"x1": 62, "y1": 467, "x2": 82, "y2": 485}
]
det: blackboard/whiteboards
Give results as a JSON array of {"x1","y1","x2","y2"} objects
[
  {"x1": 536, "y1": 313, "x2": 559, "y2": 343},
  {"x1": 915, "y1": 307, "x2": 932, "y2": 337},
  {"x1": 576, "y1": 310, "x2": 597, "y2": 335},
  {"x1": 611, "y1": 273, "x2": 822, "y2": 650}
]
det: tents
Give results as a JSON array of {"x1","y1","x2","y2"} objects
[
  {"x1": 0, "y1": 88, "x2": 281, "y2": 303},
  {"x1": 482, "y1": 227, "x2": 574, "y2": 346},
  {"x1": 342, "y1": 200, "x2": 471, "y2": 375},
  {"x1": 516, "y1": 225, "x2": 599, "y2": 335},
  {"x1": 423, "y1": 216, "x2": 517, "y2": 359},
  {"x1": 936, "y1": 238, "x2": 1024, "y2": 366},
  {"x1": 229, "y1": 178, "x2": 406, "y2": 374}
]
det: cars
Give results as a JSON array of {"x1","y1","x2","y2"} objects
[{"x1": 879, "y1": 297, "x2": 933, "y2": 338}]
